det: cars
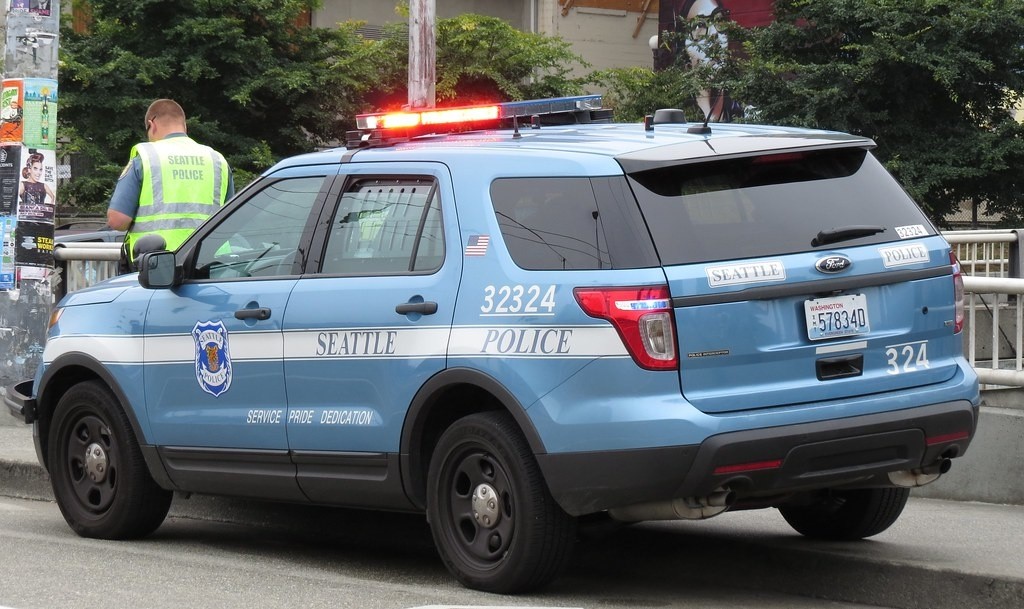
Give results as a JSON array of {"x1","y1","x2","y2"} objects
[{"x1": 54, "y1": 219, "x2": 128, "y2": 287}]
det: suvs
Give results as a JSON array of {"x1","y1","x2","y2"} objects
[{"x1": 2, "y1": 94, "x2": 981, "y2": 595}]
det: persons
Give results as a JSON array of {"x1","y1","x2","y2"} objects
[
  {"x1": 105, "y1": 98, "x2": 237, "y2": 276},
  {"x1": 19, "y1": 152, "x2": 55, "y2": 206}
]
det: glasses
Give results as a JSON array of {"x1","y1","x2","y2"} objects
[{"x1": 145, "y1": 116, "x2": 156, "y2": 138}]
